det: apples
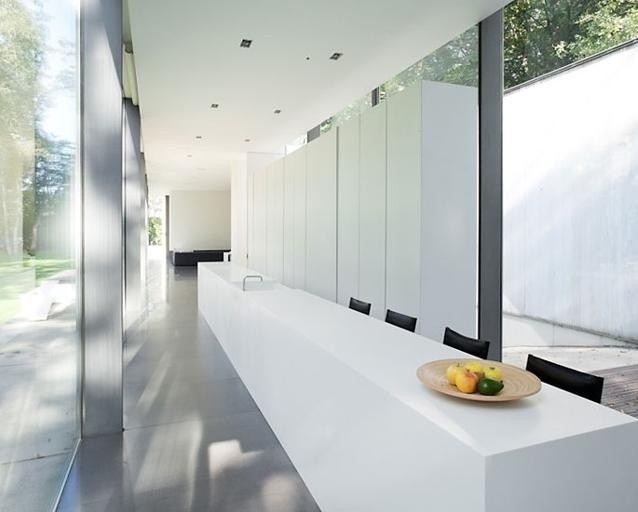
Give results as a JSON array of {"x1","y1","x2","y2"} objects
[{"x1": 447, "y1": 360, "x2": 503, "y2": 393}]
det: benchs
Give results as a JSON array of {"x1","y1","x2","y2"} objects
[{"x1": 173, "y1": 250, "x2": 231, "y2": 266}]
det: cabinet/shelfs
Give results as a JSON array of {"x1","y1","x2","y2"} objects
[{"x1": 252, "y1": 80, "x2": 478, "y2": 344}]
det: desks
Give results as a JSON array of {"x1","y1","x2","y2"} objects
[{"x1": 197, "y1": 261, "x2": 638, "y2": 512}]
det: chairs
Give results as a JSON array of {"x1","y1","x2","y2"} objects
[{"x1": 442, "y1": 327, "x2": 604, "y2": 404}]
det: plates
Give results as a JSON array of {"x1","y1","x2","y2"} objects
[{"x1": 416, "y1": 357, "x2": 543, "y2": 402}]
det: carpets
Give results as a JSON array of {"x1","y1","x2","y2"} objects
[{"x1": 588, "y1": 364, "x2": 638, "y2": 418}]
